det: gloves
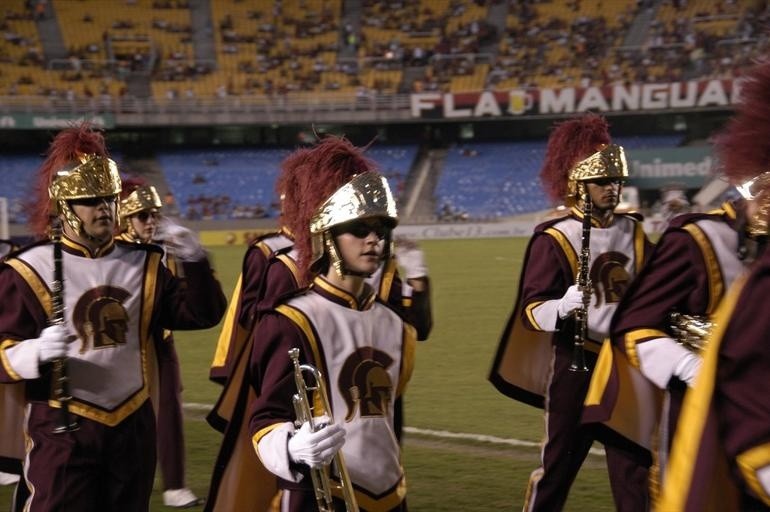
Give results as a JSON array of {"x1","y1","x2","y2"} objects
[
  {"x1": 164, "y1": 223, "x2": 204, "y2": 263},
  {"x1": 558, "y1": 284, "x2": 596, "y2": 320},
  {"x1": 673, "y1": 353, "x2": 706, "y2": 389},
  {"x1": 395, "y1": 246, "x2": 429, "y2": 286},
  {"x1": 288, "y1": 415, "x2": 347, "y2": 471},
  {"x1": 38, "y1": 324, "x2": 78, "y2": 363}
]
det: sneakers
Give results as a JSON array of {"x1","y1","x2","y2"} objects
[{"x1": 162, "y1": 487, "x2": 207, "y2": 508}]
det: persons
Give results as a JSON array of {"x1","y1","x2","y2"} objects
[
  {"x1": 437, "y1": 205, "x2": 468, "y2": 221},
  {"x1": 459, "y1": 146, "x2": 477, "y2": 157},
  {"x1": 0, "y1": 0, "x2": 770, "y2": 114},
  {"x1": 1, "y1": 122, "x2": 434, "y2": 510},
  {"x1": 517, "y1": 64, "x2": 770, "y2": 512}
]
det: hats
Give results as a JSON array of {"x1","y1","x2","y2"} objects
[
  {"x1": 112, "y1": 175, "x2": 164, "y2": 242},
  {"x1": 278, "y1": 135, "x2": 400, "y2": 282},
  {"x1": 541, "y1": 113, "x2": 629, "y2": 206},
  {"x1": 30, "y1": 123, "x2": 125, "y2": 246}
]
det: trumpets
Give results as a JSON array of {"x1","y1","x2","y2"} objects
[{"x1": 287, "y1": 348, "x2": 360, "y2": 512}]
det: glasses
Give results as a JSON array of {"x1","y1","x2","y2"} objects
[
  {"x1": 130, "y1": 211, "x2": 160, "y2": 220},
  {"x1": 331, "y1": 218, "x2": 396, "y2": 238}
]
